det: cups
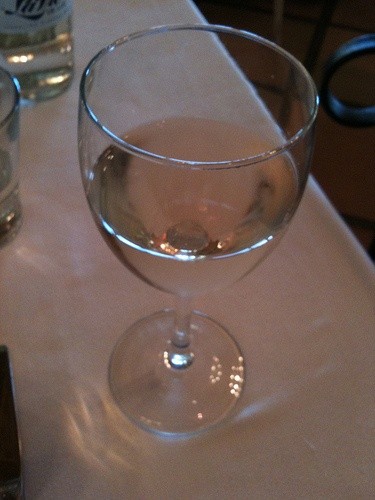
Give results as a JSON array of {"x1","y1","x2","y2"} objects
[{"x1": 0, "y1": 65, "x2": 24, "y2": 247}]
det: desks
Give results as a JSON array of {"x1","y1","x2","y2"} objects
[{"x1": 0, "y1": 2, "x2": 374, "y2": 499}]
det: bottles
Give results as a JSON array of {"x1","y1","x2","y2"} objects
[{"x1": 1, "y1": 0, "x2": 77, "y2": 102}]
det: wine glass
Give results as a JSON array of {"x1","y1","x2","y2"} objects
[{"x1": 74, "y1": 22, "x2": 318, "y2": 438}]
